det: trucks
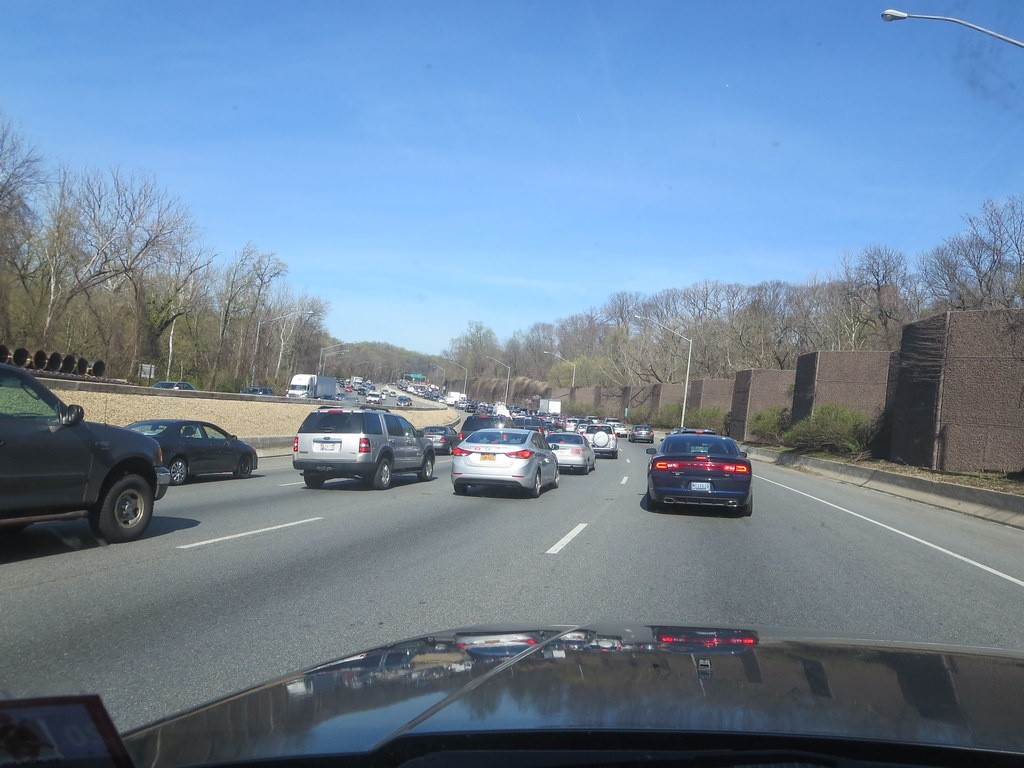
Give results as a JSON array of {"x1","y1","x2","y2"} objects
[{"x1": 285, "y1": 374, "x2": 336, "y2": 400}]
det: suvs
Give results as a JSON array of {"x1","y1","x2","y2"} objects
[
  {"x1": 0, "y1": 363, "x2": 172, "y2": 544},
  {"x1": 583, "y1": 424, "x2": 621, "y2": 460},
  {"x1": 459, "y1": 413, "x2": 517, "y2": 443},
  {"x1": 292, "y1": 405, "x2": 436, "y2": 490}
]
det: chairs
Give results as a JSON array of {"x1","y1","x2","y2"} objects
[
  {"x1": 479, "y1": 437, "x2": 489, "y2": 443},
  {"x1": 151, "y1": 425, "x2": 159, "y2": 430},
  {"x1": 510, "y1": 438, "x2": 522, "y2": 443},
  {"x1": 670, "y1": 442, "x2": 689, "y2": 452},
  {"x1": 183, "y1": 427, "x2": 194, "y2": 438},
  {"x1": 710, "y1": 445, "x2": 726, "y2": 455}
]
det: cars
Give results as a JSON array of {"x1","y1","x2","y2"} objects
[
  {"x1": 395, "y1": 379, "x2": 721, "y2": 443},
  {"x1": 1, "y1": 625, "x2": 1024, "y2": 768},
  {"x1": 646, "y1": 433, "x2": 753, "y2": 517},
  {"x1": 543, "y1": 432, "x2": 597, "y2": 476},
  {"x1": 121, "y1": 419, "x2": 259, "y2": 487},
  {"x1": 239, "y1": 387, "x2": 275, "y2": 396},
  {"x1": 420, "y1": 425, "x2": 460, "y2": 455},
  {"x1": 451, "y1": 428, "x2": 560, "y2": 498},
  {"x1": 317, "y1": 374, "x2": 413, "y2": 407},
  {"x1": 149, "y1": 381, "x2": 197, "y2": 391}
]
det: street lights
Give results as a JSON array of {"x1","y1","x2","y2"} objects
[
  {"x1": 317, "y1": 342, "x2": 403, "y2": 385},
  {"x1": 543, "y1": 351, "x2": 576, "y2": 388},
  {"x1": 448, "y1": 360, "x2": 468, "y2": 394},
  {"x1": 634, "y1": 315, "x2": 693, "y2": 427},
  {"x1": 251, "y1": 311, "x2": 314, "y2": 386},
  {"x1": 429, "y1": 362, "x2": 446, "y2": 385},
  {"x1": 486, "y1": 356, "x2": 511, "y2": 406}
]
